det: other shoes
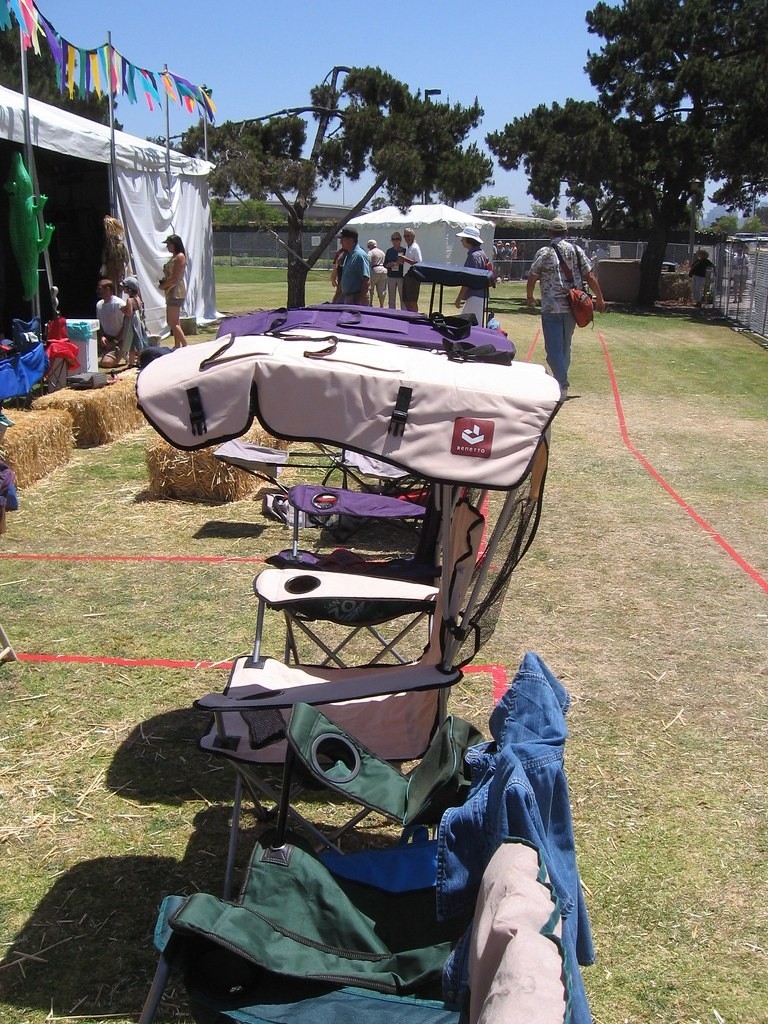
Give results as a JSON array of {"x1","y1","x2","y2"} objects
[
  {"x1": 734, "y1": 298, "x2": 741, "y2": 303},
  {"x1": 694, "y1": 303, "x2": 702, "y2": 308}
]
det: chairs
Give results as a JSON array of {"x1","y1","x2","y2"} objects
[
  {"x1": 134, "y1": 259, "x2": 598, "y2": 1024},
  {"x1": 0, "y1": 318, "x2": 79, "y2": 411}
]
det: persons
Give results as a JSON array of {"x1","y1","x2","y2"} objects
[
  {"x1": 93, "y1": 279, "x2": 129, "y2": 368},
  {"x1": 118, "y1": 277, "x2": 145, "y2": 368},
  {"x1": 455, "y1": 227, "x2": 490, "y2": 328},
  {"x1": 491, "y1": 240, "x2": 526, "y2": 280},
  {"x1": 526, "y1": 218, "x2": 605, "y2": 391},
  {"x1": 681, "y1": 246, "x2": 751, "y2": 307},
  {"x1": 159, "y1": 235, "x2": 189, "y2": 351},
  {"x1": 331, "y1": 227, "x2": 422, "y2": 313}
]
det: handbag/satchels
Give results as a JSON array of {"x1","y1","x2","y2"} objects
[
  {"x1": 689, "y1": 271, "x2": 692, "y2": 278},
  {"x1": 567, "y1": 287, "x2": 594, "y2": 328},
  {"x1": 485, "y1": 259, "x2": 497, "y2": 286}
]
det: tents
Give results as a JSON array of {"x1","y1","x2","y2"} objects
[
  {"x1": 0, "y1": 83, "x2": 225, "y2": 345},
  {"x1": 341, "y1": 204, "x2": 495, "y2": 269}
]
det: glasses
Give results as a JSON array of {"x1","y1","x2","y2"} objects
[
  {"x1": 404, "y1": 235, "x2": 412, "y2": 237},
  {"x1": 392, "y1": 238, "x2": 400, "y2": 241}
]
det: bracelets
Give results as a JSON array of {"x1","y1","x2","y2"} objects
[
  {"x1": 528, "y1": 297, "x2": 534, "y2": 299},
  {"x1": 114, "y1": 339, "x2": 119, "y2": 344}
]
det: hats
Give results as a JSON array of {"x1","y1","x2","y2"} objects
[
  {"x1": 549, "y1": 218, "x2": 566, "y2": 232},
  {"x1": 696, "y1": 249, "x2": 710, "y2": 258},
  {"x1": 337, "y1": 227, "x2": 358, "y2": 238},
  {"x1": 120, "y1": 276, "x2": 140, "y2": 291},
  {"x1": 162, "y1": 234, "x2": 180, "y2": 244},
  {"x1": 456, "y1": 227, "x2": 482, "y2": 244}
]
